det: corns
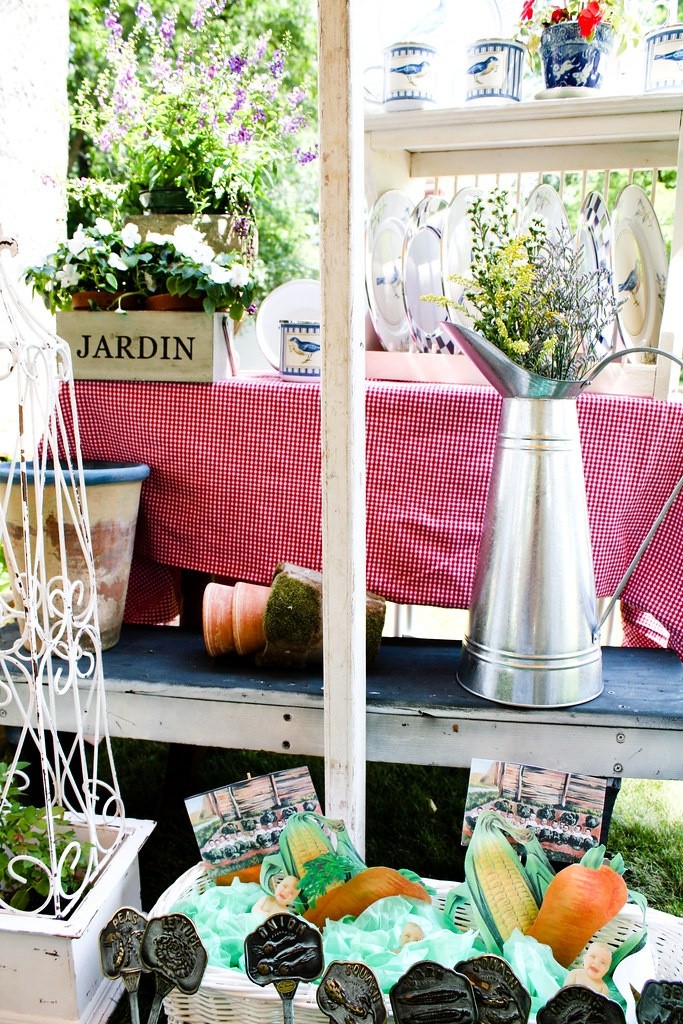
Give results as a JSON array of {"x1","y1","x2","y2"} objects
[
  {"x1": 470, "y1": 812, "x2": 540, "y2": 943},
  {"x1": 282, "y1": 813, "x2": 345, "y2": 903}
]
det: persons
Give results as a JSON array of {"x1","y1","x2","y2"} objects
[
  {"x1": 251, "y1": 875, "x2": 301, "y2": 916},
  {"x1": 564, "y1": 942, "x2": 613, "y2": 997},
  {"x1": 472, "y1": 807, "x2": 594, "y2": 844},
  {"x1": 207, "y1": 819, "x2": 290, "y2": 850},
  {"x1": 393, "y1": 922, "x2": 426, "y2": 954}
]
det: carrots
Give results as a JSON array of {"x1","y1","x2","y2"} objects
[
  {"x1": 300, "y1": 866, "x2": 430, "y2": 932},
  {"x1": 525, "y1": 845, "x2": 627, "y2": 970}
]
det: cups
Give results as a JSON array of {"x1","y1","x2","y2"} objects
[
  {"x1": 260, "y1": 562, "x2": 387, "y2": 666},
  {"x1": 280, "y1": 324, "x2": 322, "y2": 382},
  {"x1": 231, "y1": 581, "x2": 272, "y2": 655},
  {"x1": 464, "y1": 37, "x2": 526, "y2": 106},
  {"x1": 362, "y1": 41, "x2": 437, "y2": 112},
  {"x1": 0, "y1": 460, "x2": 150, "y2": 653},
  {"x1": 642, "y1": 24, "x2": 683, "y2": 95},
  {"x1": 203, "y1": 582, "x2": 233, "y2": 656}
]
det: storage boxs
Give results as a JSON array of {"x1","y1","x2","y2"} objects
[{"x1": 53, "y1": 216, "x2": 262, "y2": 387}]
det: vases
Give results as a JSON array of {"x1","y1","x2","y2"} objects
[
  {"x1": 0, "y1": 459, "x2": 152, "y2": 656},
  {"x1": 280, "y1": 321, "x2": 322, "y2": 385},
  {"x1": 533, "y1": 20, "x2": 616, "y2": 99},
  {"x1": 460, "y1": 36, "x2": 527, "y2": 107},
  {"x1": 202, "y1": 582, "x2": 234, "y2": 659},
  {"x1": 362, "y1": 42, "x2": 438, "y2": 112},
  {"x1": 232, "y1": 582, "x2": 271, "y2": 655},
  {"x1": 138, "y1": 184, "x2": 232, "y2": 217},
  {"x1": 443, "y1": 319, "x2": 683, "y2": 708},
  {"x1": 642, "y1": 24, "x2": 683, "y2": 93}
]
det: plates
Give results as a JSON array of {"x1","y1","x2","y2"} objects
[
  {"x1": 255, "y1": 278, "x2": 323, "y2": 370},
  {"x1": 363, "y1": 187, "x2": 667, "y2": 364}
]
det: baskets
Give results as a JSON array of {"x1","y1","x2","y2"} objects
[{"x1": 145, "y1": 859, "x2": 683, "y2": 1024}]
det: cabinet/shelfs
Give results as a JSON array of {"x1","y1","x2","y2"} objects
[
  {"x1": 362, "y1": 92, "x2": 683, "y2": 402},
  {"x1": 0, "y1": 0, "x2": 683, "y2": 854}
]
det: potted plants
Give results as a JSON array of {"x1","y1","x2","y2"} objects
[{"x1": 0, "y1": 760, "x2": 158, "y2": 1024}]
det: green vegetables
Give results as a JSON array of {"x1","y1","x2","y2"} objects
[{"x1": 295, "y1": 853, "x2": 369, "y2": 912}]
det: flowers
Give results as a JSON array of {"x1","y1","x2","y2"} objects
[
  {"x1": 17, "y1": 214, "x2": 268, "y2": 324},
  {"x1": 514, "y1": 0, "x2": 642, "y2": 76},
  {"x1": 67, "y1": 0, "x2": 321, "y2": 321},
  {"x1": 421, "y1": 184, "x2": 632, "y2": 384}
]
